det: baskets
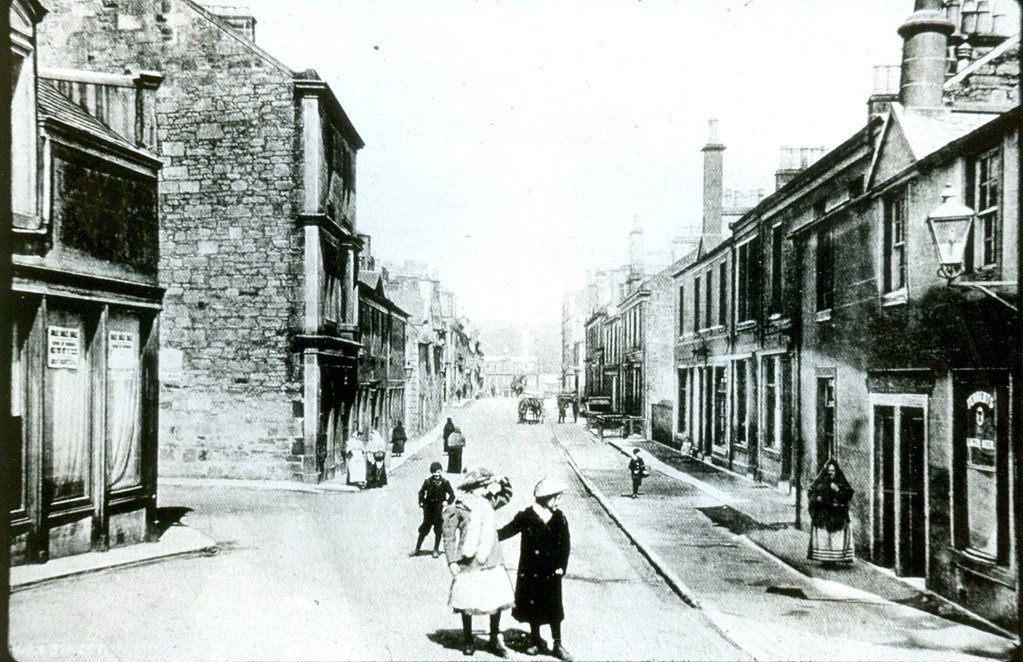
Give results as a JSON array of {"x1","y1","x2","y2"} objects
[{"x1": 641, "y1": 464, "x2": 652, "y2": 477}]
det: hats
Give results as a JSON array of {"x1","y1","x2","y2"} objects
[
  {"x1": 457, "y1": 466, "x2": 497, "y2": 489},
  {"x1": 534, "y1": 474, "x2": 563, "y2": 496}
]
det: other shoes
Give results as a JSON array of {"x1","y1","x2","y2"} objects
[
  {"x1": 431, "y1": 549, "x2": 439, "y2": 558},
  {"x1": 551, "y1": 642, "x2": 571, "y2": 661},
  {"x1": 463, "y1": 636, "x2": 474, "y2": 655},
  {"x1": 408, "y1": 550, "x2": 418, "y2": 556},
  {"x1": 527, "y1": 638, "x2": 548, "y2": 654},
  {"x1": 486, "y1": 637, "x2": 507, "y2": 658}
]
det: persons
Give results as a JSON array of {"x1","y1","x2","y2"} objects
[
  {"x1": 390, "y1": 422, "x2": 407, "y2": 456},
  {"x1": 497, "y1": 476, "x2": 572, "y2": 660},
  {"x1": 409, "y1": 462, "x2": 453, "y2": 558},
  {"x1": 446, "y1": 427, "x2": 465, "y2": 472},
  {"x1": 444, "y1": 418, "x2": 455, "y2": 449},
  {"x1": 573, "y1": 398, "x2": 579, "y2": 423},
  {"x1": 463, "y1": 384, "x2": 467, "y2": 398},
  {"x1": 558, "y1": 399, "x2": 567, "y2": 423},
  {"x1": 443, "y1": 469, "x2": 517, "y2": 656},
  {"x1": 455, "y1": 389, "x2": 462, "y2": 400},
  {"x1": 629, "y1": 449, "x2": 648, "y2": 498},
  {"x1": 346, "y1": 431, "x2": 387, "y2": 489},
  {"x1": 808, "y1": 460, "x2": 856, "y2": 564}
]
